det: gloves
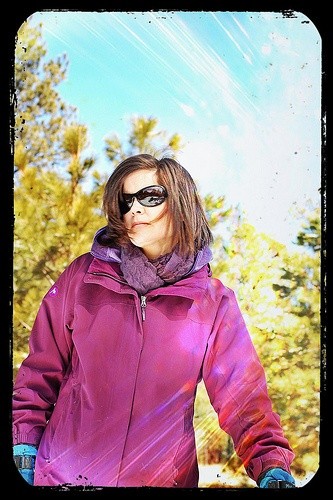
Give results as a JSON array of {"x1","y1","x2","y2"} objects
[
  {"x1": 258, "y1": 468, "x2": 295, "y2": 488},
  {"x1": 13, "y1": 443, "x2": 38, "y2": 485}
]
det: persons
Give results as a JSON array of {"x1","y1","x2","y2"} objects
[{"x1": 13, "y1": 154, "x2": 296, "y2": 490}]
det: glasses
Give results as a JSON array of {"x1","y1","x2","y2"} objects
[{"x1": 119, "y1": 184, "x2": 170, "y2": 215}]
